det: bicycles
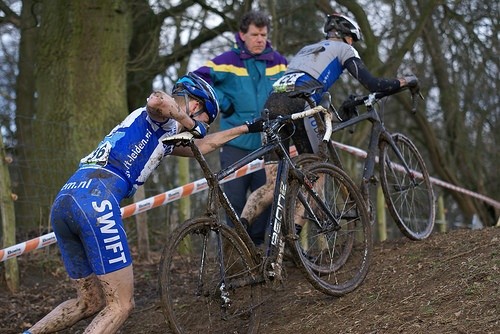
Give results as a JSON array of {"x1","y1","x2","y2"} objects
[
  {"x1": 284, "y1": 73, "x2": 438, "y2": 276},
  {"x1": 159, "y1": 92, "x2": 375, "y2": 334}
]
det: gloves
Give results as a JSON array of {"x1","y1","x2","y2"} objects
[
  {"x1": 404, "y1": 75, "x2": 420, "y2": 94},
  {"x1": 243, "y1": 117, "x2": 268, "y2": 134},
  {"x1": 336, "y1": 110, "x2": 351, "y2": 122},
  {"x1": 188, "y1": 121, "x2": 209, "y2": 140}
]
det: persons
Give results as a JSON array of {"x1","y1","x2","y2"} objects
[
  {"x1": 189, "y1": 14, "x2": 290, "y2": 257},
  {"x1": 239, "y1": 13, "x2": 419, "y2": 236},
  {"x1": 22, "y1": 72, "x2": 272, "y2": 334}
]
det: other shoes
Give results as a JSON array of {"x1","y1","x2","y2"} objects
[{"x1": 282, "y1": 248, "x2": 319, "y2": 266}]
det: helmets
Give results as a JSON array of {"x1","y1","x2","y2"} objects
[
  {"x1": 172, "y1": 72, "x2": 219, "y2": 126},
  {"x1": 324, "y1": 14, "x2": 361, "y2": 42}
]
